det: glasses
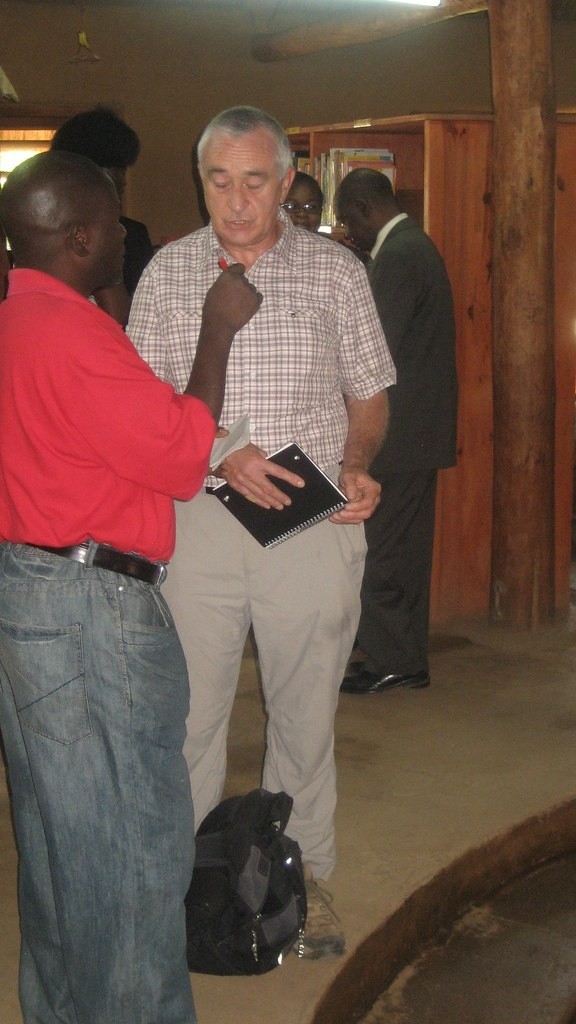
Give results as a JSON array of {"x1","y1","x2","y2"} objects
[{"x1": 281, "y1": 204, "x2": 324, "y2": 215}]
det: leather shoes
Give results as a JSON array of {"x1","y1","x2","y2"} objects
[{"x1": 338, "y1": 670, "x2": 431, "y2": 692}]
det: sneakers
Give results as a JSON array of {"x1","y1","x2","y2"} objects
[{"x1": 293, "y1": 866, "x2": 347, "y2": 959}]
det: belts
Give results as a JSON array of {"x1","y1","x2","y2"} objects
[{"x1": 25, "y1": 539, "x2": 167, "y2": 587}]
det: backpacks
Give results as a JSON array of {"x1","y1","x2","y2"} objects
[{"x1": 185, "y1": 790, "x2": 307, "y2": 976}]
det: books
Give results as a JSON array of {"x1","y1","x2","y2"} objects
[
  {"x1": 297, "y1": 149, "x2": 396, "y2": 226},
  {"x1": 212, "y1": 443, "x2": 349, "y2": 550}
]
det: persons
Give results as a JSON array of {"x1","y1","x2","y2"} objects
[
  {"x1": 332, "y1": 168, "x2": 457, "y2": 697},
  {"x1": 123, "y1": 106, "x2": 392, "y2": 959},
  {"x1": 281, "y1": 170, "x2": 322, "y2": 232},
  {"x1": 43, "y1": 104, "x2": 156, "y2": 332},
  {"x1": 0, "y1": 150, "x2": 264, "y2": 1024}
]
